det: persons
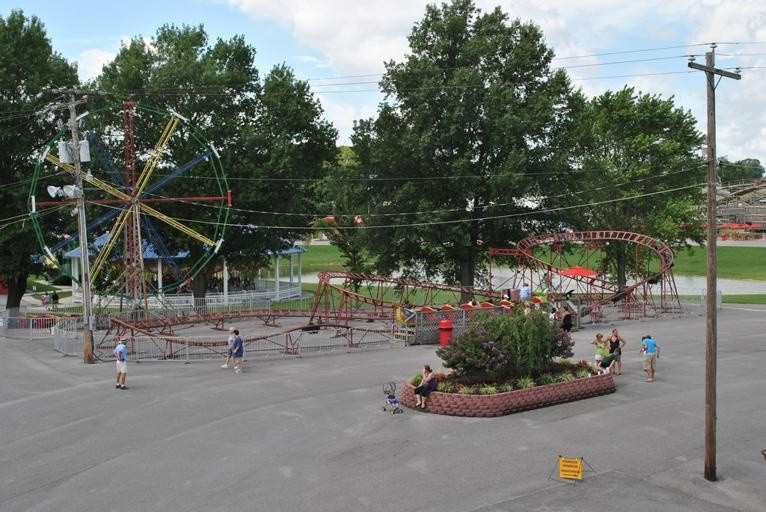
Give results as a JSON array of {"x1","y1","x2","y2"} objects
[
  {"x1": 560, "y1": 307, "x2": 572, "y2": 332},
  {"x1": 404, "y1": 299, "x2": 410, "y2": 309},
  {"x1": 590, "y1": 332, "x2": 607, "y2": 367},
  {"x1": 519, "y1": 283, "x2": 531, "y2": 303},
  {"x1": 604, "y1": 328, "x2": 626, "y2": 375},
  {"x1": 231, "y1": 329, "x2": 243, "y2": 373},
  {"x1": 113, "y1": 335, "x2": 129, "y2": 390},
  {"x1": 43, "y1": 292, "x2": 50, "y2": 310},
  {"x1": 469, "y1": 296, "x2": 476, "y2": 305},
  {"x1": 503, "y1": 294, "x2": 510, "y2": 301},
  {"x1": 385, "y1": 389, "x2": 400, "y2": 408},
  {"x1": 52, "y1": 291, "x2": 58, "y2": 310},
  {"x1": 599, "y1": 347, "x2": 620, "y2": 374},
  {"x1": 524, "y1": 303, "x2": 531, "y2": 317},
  {"x1": 414, "y1": 364, "x2": 437, "y2": 409},
  {"x1": 407, "y1": 302, "x2": 417, "y2": 314},
  {"x1": 220, "y1": 326, "x2": 236, "y2": 368},
  {"x1": 470, "y1": 300, "x2": 481, "y2": 307},
  {"x1": 640, "y1": 335, "x2": 660, "y2": 382},
  {"x1": 641, "y1": 335, "x2": 656, "y2": 372},
  {"x1": 549, "y1": 308, "x2": 558, "y2": 326}
]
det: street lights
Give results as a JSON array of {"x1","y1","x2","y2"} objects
[{"x1": 73, "y1": 164, "x2": 95, "y2": 364}]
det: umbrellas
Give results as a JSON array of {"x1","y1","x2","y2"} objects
[{"x1": 559, "y1": 263, "x2": 598, "y2": 293}]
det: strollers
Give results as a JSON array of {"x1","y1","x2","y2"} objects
[{"x1": 382, "y1": 381, "x2": 403, "y2": 415}]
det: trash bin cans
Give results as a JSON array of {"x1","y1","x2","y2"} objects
[{"x1": 437, "y1": 320, "x2": 455, "y2": 346}]
